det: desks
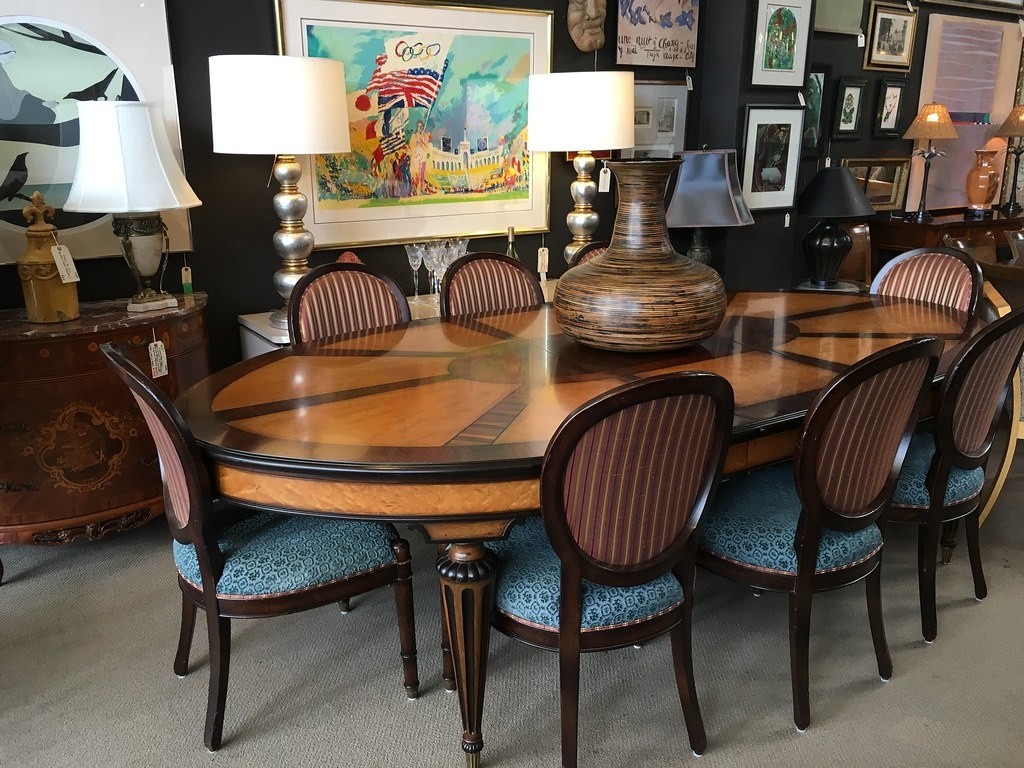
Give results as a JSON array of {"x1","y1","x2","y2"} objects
[
  {"x1": 0, "y1": 290, "x2": 213, "y2": 587},
  {"x1": 876, "y1": 212, "x2": 1024, "y2": 254},
  {"x1": 103, "y1": 289, "x2": 989, "y2": 765}
]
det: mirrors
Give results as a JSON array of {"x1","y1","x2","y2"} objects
[{"x1": 0, "y1": 19, "x2": 148, "y2": 234}]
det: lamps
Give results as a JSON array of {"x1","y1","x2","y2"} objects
[
  {"x1": 63, "y1": 100, "x2": 204, "y2": 314},
  {"x1": 662, "y1": 142, "x2": 757, "y2": 270},
  {"x1": 527, "y1": 68, "x2": 635, "y2": 265},
  {"x1": 206, "y1": 55, "x2": 349, "y2": 331},
  {"x1": 779, "y1": 165, "x2": 873, "y2": 285},
  {"x1": 988, "y1": 104, "x2": 1024, "y2": 213},
  {"x1": 901, "y1": 102, "x2": 959, "y2": 224}
]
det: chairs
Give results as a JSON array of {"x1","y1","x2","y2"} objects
[
  {"x1": 686, "y1": 333, "x2": 946, "y2": 731},
  {"x1": 440, "y1": 367, "x2": 735, "y2": 766},
  {"x1": 98, "y1": 344, "x2": 421, "y2": 751},
  {"x1": 567, "y1": 241, "x2": 607, "y2": 274},
  {"x1": 941, "y1": 231, "x2": 997, "y2": 264},
  {"x1": 1003, "y1": 228, "x2": 1024, "y2": 260},
  {"x1": 855, "y1": 308, "x2": 1024, "y2": 643},
  {"x1": 287, "y1": 262, "x2": 416, "y2": 349},
  {"x1": 869, "y1": 249, "x2": 983, "y2": 315},
  {"x1": 440, "y1": 253, "x2": 543, "y2": 321}
]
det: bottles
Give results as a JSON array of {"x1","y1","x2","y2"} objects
[
  {"x1": 17, "y1": 190, "x2": 80, "y2": 323},
  {"x1": 966, "y1": 150, "x2": 1000, "y2": 210},
  {"x1": 505, "y1": 227, "x2": 521, "y2": 263},
  {"x1": 553, "y1": 157, "x2": 727, "y2": 352}
]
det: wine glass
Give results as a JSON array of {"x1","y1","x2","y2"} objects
[{"x1": 404, "y1": 238, "x2": 469, "y2": 304}]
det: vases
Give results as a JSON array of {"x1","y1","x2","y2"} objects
[
  {"x1": 552, "y1": 158, "x2": 727, "y2": 352},
  {"x1": 965, "y1": 149, "x2": 999, "y2": 212}
]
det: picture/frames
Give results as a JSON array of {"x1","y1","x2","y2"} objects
[
  {"x1": 801, "y1": 63, "x2": 834, "y2": 160},
  {"x1": 603, "y1": 0, "x2": 706, "y2": 79},
  {"x1": 842, "y1": 152, "x2": 913, "y2": 214},
  {"x1": 566, "y1": 149, "x2": 613, "y2": 162},
  {"x1": 618, "y1": 79, "x2": 699, "y2": 161},
  {"x1": 872, "y1": 76, "x2": 911, "y2": 139},
  {"x1": 274, "y1": 0, "x2": 556, "y2": 250},
  {"x1": 733, "y1": 102, "x2": 807, "y2": 210},
  {"x1": 861, "y1": 1, "x2": 921, "y2": 74},
  {"x1": 831, "y1": 78, "x2": 868, "y2": 140},
  {"x1": 741, "y1": 1, "x2": 817, "y2": 92}
]
